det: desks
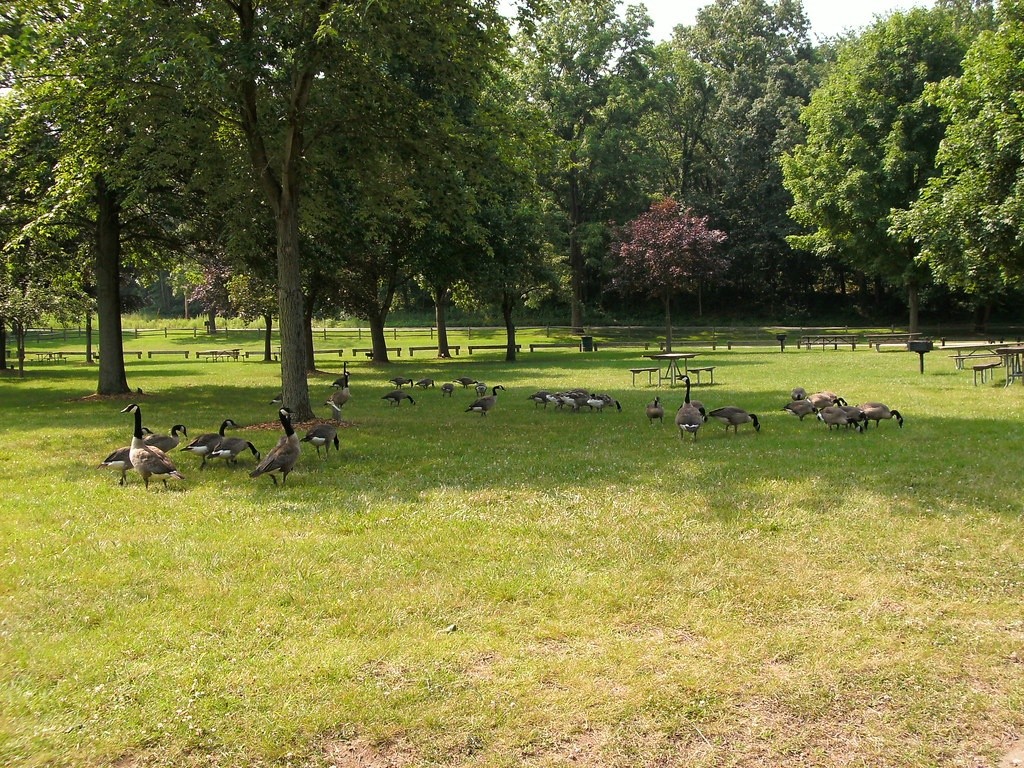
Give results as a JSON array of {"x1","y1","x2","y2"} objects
[
  {"x1": 210, "y1": 351, "x2": 239, "y2": 362},
  {"x1": 802, "y1": 333, "x2": 859, "y2": 350},
  {"x1": 643, "y1": 352, "x2": 700, "y2": 387},
  {"x1": 468, "y1": 345, "x2": 521, "y2": 355},
  {"x1": 863, "y1": 333, "x2": 921, "y2": 348},
  {"x1": 938, "y1": 341, "x2": 1022, "y2": 368},
  {"x1": 996, "y1": 347, "x2": 1024, "y2": 388}
]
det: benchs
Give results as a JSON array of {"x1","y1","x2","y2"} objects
[
  {"x1": 948, "y1": 351, "x2": 997, "y2": 360},
  {"x1": 5, "y1": 349, "x2": 12, "y2": 357},
  {"x1": 972, "y1": 362, "x2": 1002, "y2": 371},
  {"x1": 148, "y1": 350, "x2": 189, "y2": 358},
  {"x1": 867, "y1": 339, "x2": 928, "y2": 344},
  {"x1": 409, "y1": 345, "x2": 460, "y2": 356},
  {"x1": 352, "y1": 347, "x2": 402, "y2": 357},
  {"x1": 529, "y1": 341, "x2": 789, "y2": 353},
  {"x1": 123, "y1": 350, "x2": 142, "y2": 359},
  {"x1": 52, "y1": 352, "x2": 97, "y2": 359},
  {"x1": 16, "y1": 350, "x2": 54, "y2": 360},
  {"x1": 796, "y1": 339, "x2": 860, "y2": 345},
  {"x1": 245, "y1": 351, "x2": 282, "y2": 359},
  {"x1": 628, "y1": 365, "x2": 717, "y2": 374},
  {"x1": 196, "y1": 351, "x2": 244, "y2": 359},
  {"x1": 314, "y1": 349, "x2": 344, "y2": 357}
]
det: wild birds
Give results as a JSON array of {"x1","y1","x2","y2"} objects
[{"x1": 95, "y1": 371, "x2": 905, "y2": 495}]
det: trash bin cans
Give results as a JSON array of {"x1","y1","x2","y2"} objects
[{"x1": 581, "y1": 336, "x2": 593, "y2": 352}]
